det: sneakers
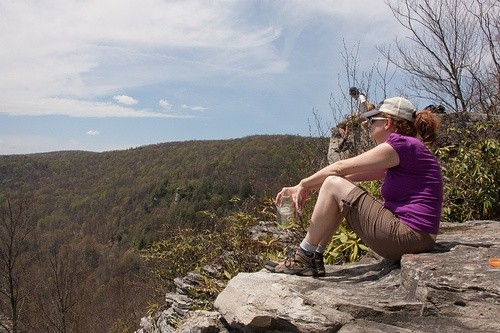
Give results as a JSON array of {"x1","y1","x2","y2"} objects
[
  {"x1": 314, "y1": 251, "x2": 325, "y2": 276},
  {"x1": 264, "y1": 251, "x2": 316, "y2": 276}
]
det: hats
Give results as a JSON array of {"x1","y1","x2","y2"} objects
[{"x1": 360, "y1": 95, "x2": 417, "y2": 122}]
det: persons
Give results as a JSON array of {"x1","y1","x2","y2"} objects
[
  {"x1": 264, "y1": 97, "x2": 442, "y2": 276},
  {"x1": 348, "y1": 87, "x2": 375, "y2": 125}
]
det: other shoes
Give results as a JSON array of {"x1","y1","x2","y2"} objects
[
  {"x1": 335, "y1": 145, "x2": 343, "y2": 153},
  {"x1": 342, "y1": 141, "x2": 352, "y2": 152}
]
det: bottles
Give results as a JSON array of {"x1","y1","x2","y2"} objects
[{"x1": 277, "y1": 193, "x2": 294, "y2": 229}]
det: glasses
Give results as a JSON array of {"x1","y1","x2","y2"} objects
[{"x1": 367, "y1": 117, "x2": 397, "y2": 125}]
